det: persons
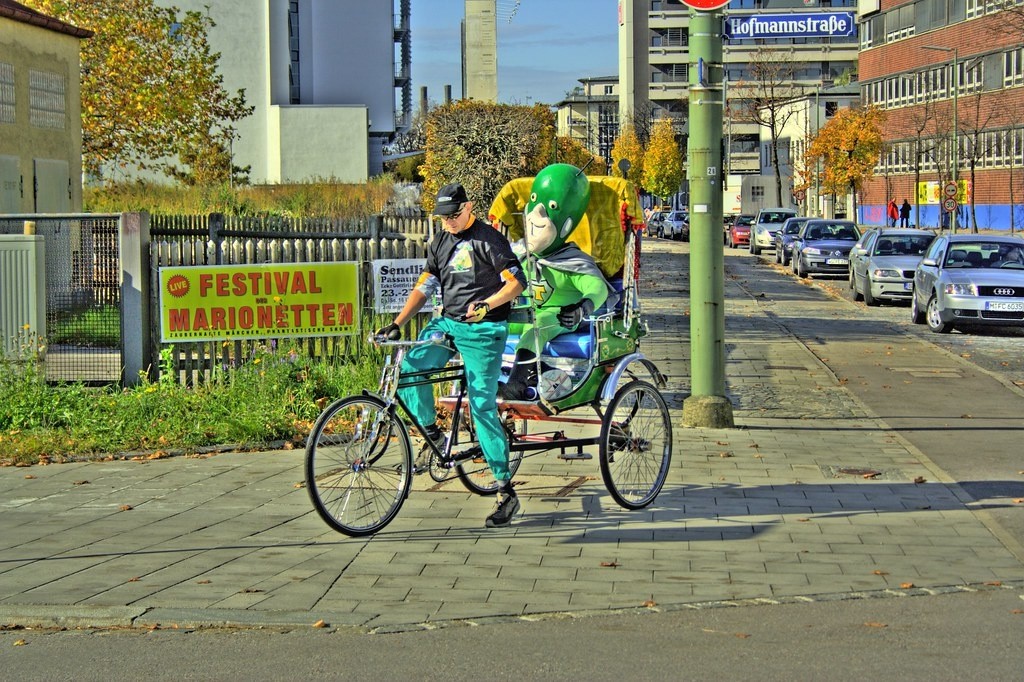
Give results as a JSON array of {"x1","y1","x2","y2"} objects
[
  {"x1": 773, "y1": 213, "x2": 785, "y2": 223},
  {"x1": 787, "y1": 224, "x2": 799, "y2": 234},
  {"x1": 888, "y1": 196, "x2": 899, "y2": 227},
  {"x1": 990, "y1": 245, "x2": 1020, "y2": 267},
  {"x1": 366, "y1": 185, "x2": 527, "y2": 528},
  {"x1": 899, "y1": 199, "x2": 911, "y2": 228},
  {"x1": 498, "y1": 163, "x2": 608, "y2": 401},
  {"x1": 762, "y1": 213, "x2": 771, "y2": 223}
]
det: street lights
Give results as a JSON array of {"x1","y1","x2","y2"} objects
[
  {"x1": 790, "y1": 82, "x2": 821, "y2": 218},
  {"x1": 922, "y1": 46, "x2": 958, "y2": 234}
]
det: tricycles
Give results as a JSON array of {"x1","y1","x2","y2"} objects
[{"x1": 304, "y1": 175, "x2": 672, "y2": 538}]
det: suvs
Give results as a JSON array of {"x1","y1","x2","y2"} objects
[
  {"x1": 852, "y1": 226, "x2": 937, "y2": 307},
  {"x1": 749, "y1": 208, "x2": 801, "y2": 255}
]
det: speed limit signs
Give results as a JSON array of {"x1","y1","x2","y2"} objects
[{"x1": 944, "y1": 198, "x2": 956, "y2": 211}]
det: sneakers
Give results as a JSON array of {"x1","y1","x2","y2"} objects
[
  {"x1": 413, "y1": 430, "x2": 447, "y2": 476},
  {"x1": 485, "y1": 492, "x2": 520, "y2": 528}
]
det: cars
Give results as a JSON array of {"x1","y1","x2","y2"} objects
[
  {"x1": 660, "y1": 211, "x2": 690, "y2": 240},
  {"x1": 910, "y1": 233, "x2": 1024, "y2": 334},
  {"x1": 791, "y1": 219, "x2": 862, "y2": 279},
  {"x1": 775, "y1": 217, "x2": 828, "y2": 266},
  {"x1": 646, "y1": 212, "x2": 671, "y2": 238},
  {"x1": 848, "y1": 228, "x2": 874, "y2": 289},
  {"x1": 680, "y1": 216, "x2": 690, "y2": 243},
  {"x1": 723, "y1": 214, "x2": 741, "y2": 246},
  {"x1": 729, "y1": 214, "x2": 756, "y2": 248}
]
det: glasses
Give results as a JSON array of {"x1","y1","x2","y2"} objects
[{"x1": 441, "y1": 211, "x2": 461, "y2": 219}]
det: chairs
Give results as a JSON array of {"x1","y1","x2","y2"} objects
[
  {"x1": 951, "y1": 250, "x2": 971, "y2": 266},
  {"x1": 783, "y1": 214, "x2": 792, "y2": 223},
  {"x1": 790, "y1": 224, "x2": 799, "y2": 233},
  {"x1": 839, "y1": 229, "x2": 849, "y2": 238},
  {"x1": 911, "y1": 242, "x2": 919, "y2": 250},
  {"x1": 990, "y1": 252, "x2": 999, "y2": 261},
  {"x1": 877, "y1": 240, "x2": 892, "y2": 251},
  {"x1": 764, "y1": 214, "x2": 771, "y2": 223},
  {"x1": 893, "y1": 242, "x2": 906, "y2": 250},
  {"x1": 811, "y1": 229, "x2": 823, "y2": 239},
  {"x1": 968, "y1": 252, "x2": 984, "y2": 266}
]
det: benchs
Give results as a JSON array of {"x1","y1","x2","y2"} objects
[{"x1": 503, "y1": 275, "x2": 637, "y2": 359}]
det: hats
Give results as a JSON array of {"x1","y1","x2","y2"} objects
[{"x1": 432, "y1": 183, "x2": 468, "y2": 215}]
